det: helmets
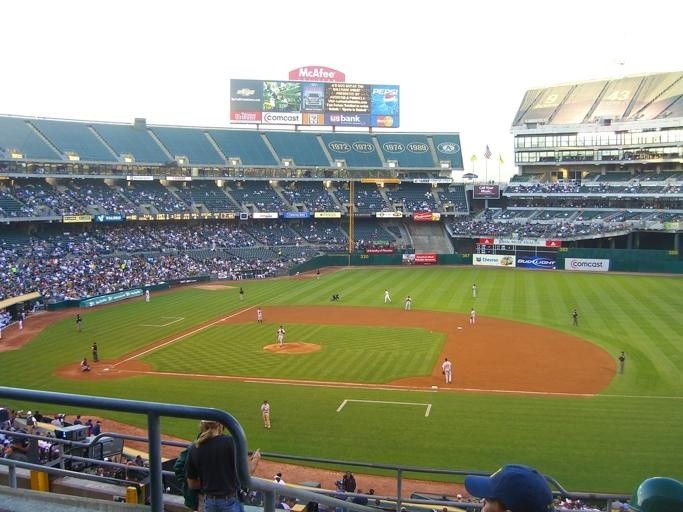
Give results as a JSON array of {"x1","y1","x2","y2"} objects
[{"x1": 627, "y1": 475, "x2": 683, "y2": 512}]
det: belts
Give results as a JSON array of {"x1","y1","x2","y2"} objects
[{"x1": 206, "y1": 492, "x2": 237, "y2": 499}]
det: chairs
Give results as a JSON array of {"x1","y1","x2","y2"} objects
[{"x1": 447, "y1": 172, "x2": 683, "y2": 242}]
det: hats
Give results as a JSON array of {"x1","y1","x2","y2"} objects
[{"x1": 461, "y1": 463, "x2": 552, "y2": 510}]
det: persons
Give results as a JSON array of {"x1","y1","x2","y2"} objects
[
  {"x1": 88, "y1": 341, "x2": 98, "y2": 363},
  {"x1": 277, "y1": 326, "x2": 285, "y2": 346},
  {"x1": 471, "y1": 284, "x2": 477, "y2": 298},
  {"x1": 17, "y1": 309, "x2": 23, "y2": 330},
  {"x1": 383, "y1": 289, "x2": 391, "y2": 303},
  {"x1": 1, "y1": 403, "x2": 682, "y2": 512},
  {"x1": 1, "y1": 155, "x2": 358, "y2": 327},
  {"x1": 468, "y1": 308, "x2": 476, "y2": 326},
  {"x1": 441, "y1": 358, "x2": 453, "y2": 384},
  {"x1": 618, "y1": 351, "x2": 625, "y2": 373},
  {"x1": 80, "y1": 357, "x2": 91, "y2": 373},
  {"x1": 259, "y1": 400, "x2": 271, "y2": 429},
  {"x1": 270, "y1": 93, "x2": 286, "y2": 110},
  {"x1": 360, "y1": 171, "x2": 683, "y2": 265},
  {"x1": 571, "y1": 308, "x2": 579, "y2": 327},
  {"x1": 74, "y1": 312, "x2": 82, "y2": 332},
  {"x1": 404, "y1": 295, "x2": 412, "y2": 310}
]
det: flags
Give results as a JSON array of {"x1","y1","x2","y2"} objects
[
  {"x1": 499, "y1": 155, "x2": 503, "y2": 164},
  {"x1": 484, "y1": 144, "x2": 492, "y2": 159},
  {"x1": 471, "y1": 152, "x2": 477, "y2": 161}
]
